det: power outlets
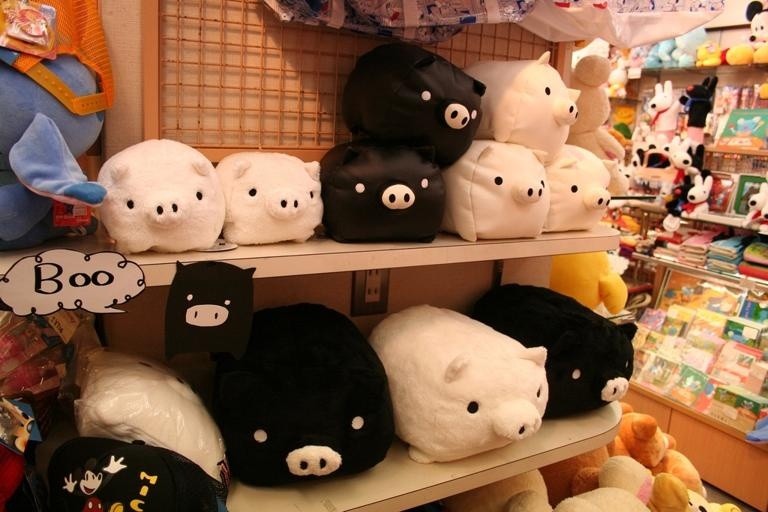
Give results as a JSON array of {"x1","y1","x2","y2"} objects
[{"x1": 352, "y1": 269, "x2": 390, "y2": 317}]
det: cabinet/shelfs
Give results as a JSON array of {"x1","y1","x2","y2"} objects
[
  {"x1": 2, "y1": 224, "x2": 623, "y2": 512},
  {"x1": 622, "y1": 54, "x2": 765, "y2": 510}
]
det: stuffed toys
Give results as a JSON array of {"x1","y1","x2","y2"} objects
[
  {"x1": 93, "y1": 139, "x2": 225, "y2": 257},
  {"x1": 0, "y1": 250, "x2": 744, "y2": 512},
  {"x1": 320, "y1": 45, "x2": 618, "y2": 245},
  {"x1": 0, "y1": 0, "x2": 767, "y2": 512},
  {"x1": 572, "y1": 1, "x2": 767, "y2": 213},
  {"x1": 2, "y1": 2, "x2": 116, "y2": 244},
  {"x1": 212, "y1": 153, "x2": 322, "y2": 244}
]
